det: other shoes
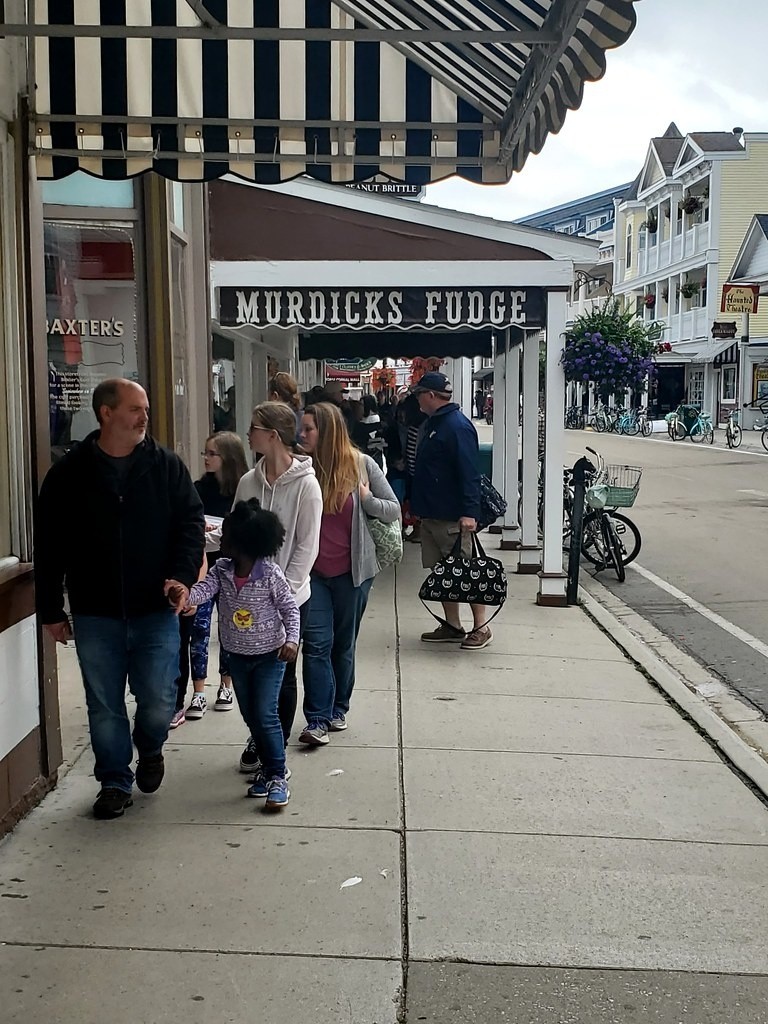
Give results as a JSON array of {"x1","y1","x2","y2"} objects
[{"x1": 406, "y1": 531, "x2": 421, "y2": 543}]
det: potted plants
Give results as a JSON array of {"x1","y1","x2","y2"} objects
[
  {"x1": 678, "y1": 193, "x2": 704, "y2": 214},
  {"x1": 644, "y1": 219, "x2": 657, "y2": 233},
  {"x1": 680, "y1": 281, "x2": 700, "y2": 299}
]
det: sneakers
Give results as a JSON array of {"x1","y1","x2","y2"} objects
[
  {"x1": 215, "y1": 683, "x2": 233, "y2": 711},
  {"x1": 246, "y1": 765, "x2": 292, "y2": 797},
  {"x1": 330, "y1": 712, "x2": 348, "y2": 730},
  {"x1": 266, "y1": 775, "x2": 291, "y2": 809},
  {"x1": 461, "y1": 625, "x2": 494, "y2": 648},
  {"x1": 134, "y1": 754, "x2": 166, "y2": 794},
  {"x1": 93, "y1": 786, "x2": 133, "y2": 819},
  {"x1": 300, "y1": 719, "x2": 330, "y2": 745},
  {"x1": 239, "y1": 735, "x2": 258, "y2": 773},
  {"x1": 170, "y1": 710, "x2": 185, "y2": 728},
  {"x1": 420, "y1": 624, "x2": 467, "y2": 643},
  {"x1": 187, "y1": 694, "x2": 207, "y2": 718}
]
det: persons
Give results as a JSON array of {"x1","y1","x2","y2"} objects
[
  {"x1": 231, "y1": 402, "x2": 324, "y2": 781},
  {"x1": 166, "y1": 497, "x2": 301, "y2": 810},
  {"x1": 31, "y1": 379, "x2": 206, "y2": 819},
  {"x1": 409, "y1": 372, "x2": 493, "y2": 649},
  {"x1": 210, "y1": 371, "x2": 428, "y2": 542},
  {"x1": 184, "y1": 430, "x2": 249, "y2": 718},
  {"x1": 298, "y1": 402, "x2": 402, "y2": 745},
  {"x1": 168, "y1": 611, "x2": 192, "y2": 729}
]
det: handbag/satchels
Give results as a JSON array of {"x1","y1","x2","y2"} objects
[
  {"x1": 418, "y1": 526, "x2": 509, "y2": 633},
  {"x1": 369, "y1": 514, "x2": 404, "y2": 570},
  {"x1": 472, "y1": 472, "x2": 507, "y2": 530}
]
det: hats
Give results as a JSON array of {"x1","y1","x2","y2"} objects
[
  {"x1": 323, "y1": 381, "x2": 350, "y2": 394},
  {"x1": 419, "y1": 371, "x2": 453, "y2": 393}
]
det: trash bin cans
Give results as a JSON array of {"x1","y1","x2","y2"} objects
[
  {"x1": 677, "y1": 404, "x2": 701, "y2": 437},
  {"x1": 479, "y1": 444, "x2": 492, "y2": 480}
]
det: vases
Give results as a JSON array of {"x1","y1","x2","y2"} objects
[{"x1": 646, "y1": 301, "x2": 655, "y2": 309}]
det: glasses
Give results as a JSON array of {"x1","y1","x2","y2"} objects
[
  {"x1": 251, "y1": 424, "x2": 282, "y2": 442},
  {"x1": 201, "y1": 451, "x2": 222, "y2": 458}
]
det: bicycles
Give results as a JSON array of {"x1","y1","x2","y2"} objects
[
  {"x1": 688, "y1": 407, "x2": 716, "y2": 446},
  {"x1": 588, "y1": 402, "x2": 654, "y2": 436},
  {"x1": 565, "y1": 403, "x2": 587, "y2": 431},
  {"x1": 665, "y1": 405, "x2": 688, "y2": 443},
  {"x1": 518, "y1": 444, "x2": 644, "y2": 583},
  {"x1": 720, "y1": 406, "x2": 743, "y2": 450},
  {"x1": 752, "y1": 415, "x2": 768, "y2": 450}
]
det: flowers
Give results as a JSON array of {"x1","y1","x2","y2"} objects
[{"x1": 643, "y1": 294, "x2": 655, "y2": 304}]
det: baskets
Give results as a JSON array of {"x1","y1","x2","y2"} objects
[{"x1": 601, "y1": 463, "x2": 644, "y2": 508}]
